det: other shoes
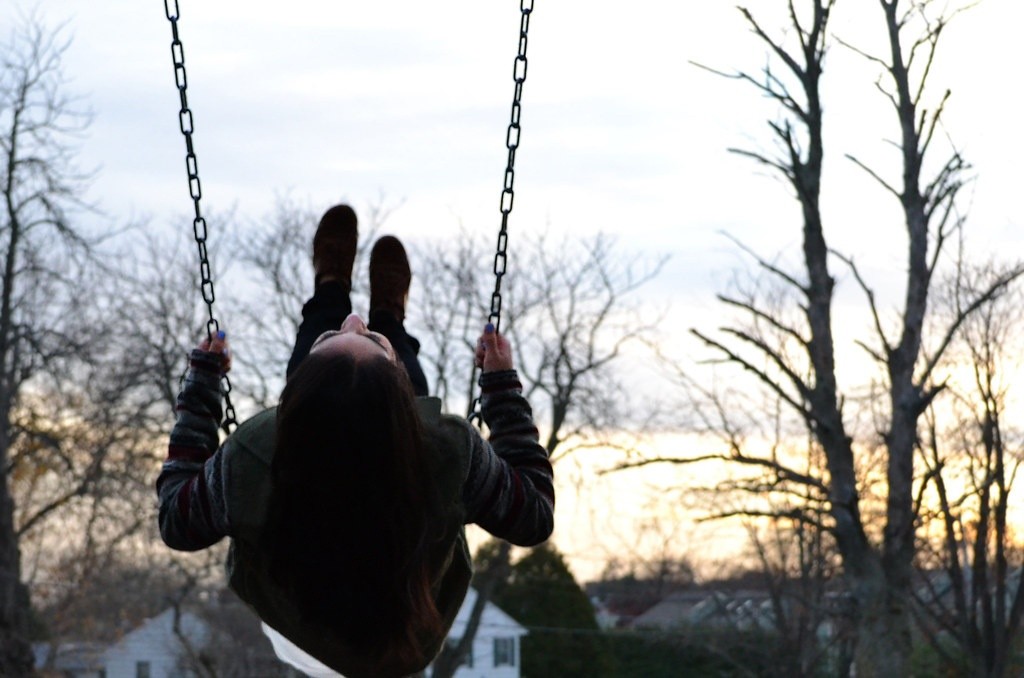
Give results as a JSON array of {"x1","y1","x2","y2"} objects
[
  {"x1": 369, "y1": 236, "x2": 411, "y2": 324},
  {"x1": 312, "y1": 205, "x2": 358, "y2": 291}
]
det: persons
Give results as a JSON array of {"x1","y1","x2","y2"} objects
[{"x1": 154, "y1": 203, "x2": 557, "y2": 678}]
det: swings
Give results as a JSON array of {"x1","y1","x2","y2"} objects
[{"x1": 160, "y1": 0, "x2": 536, "y2": 628}]
